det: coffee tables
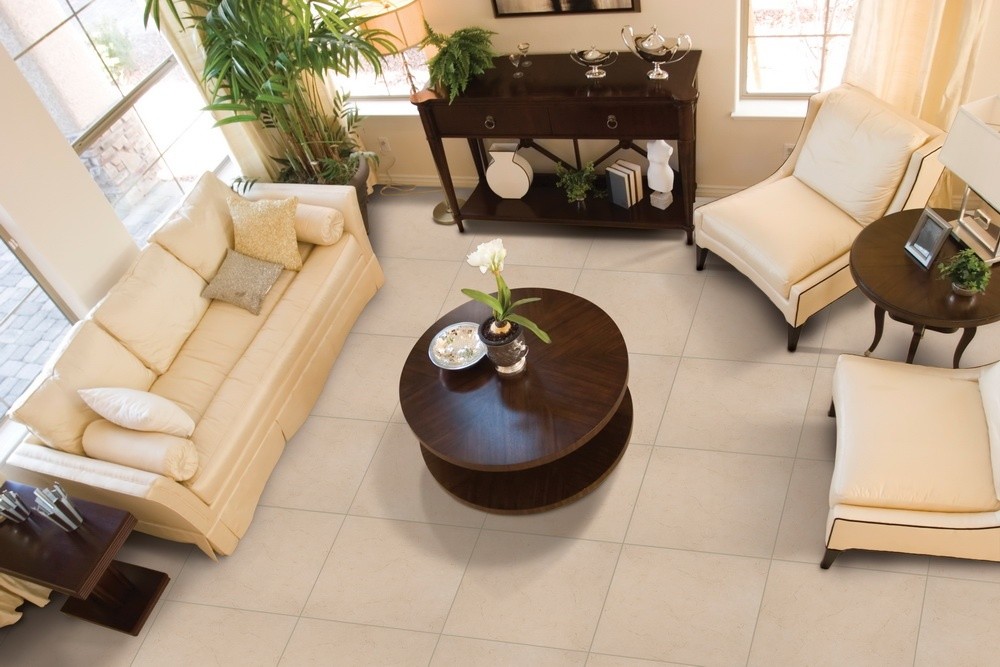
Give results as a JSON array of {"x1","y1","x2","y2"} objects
[
  {"x1": 398, "y1": 289, "x2": 635, "y2": 516},
  {"x1": 850, "y1": 209, "x2": 1000, "y2": 369}
]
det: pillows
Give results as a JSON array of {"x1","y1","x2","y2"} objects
[
  {"x1": 202, "y1": 196, "x2": 302, "y2": 315},
  {"x1": 77, "y1": 387, "x2": 195, "y2": 438}
]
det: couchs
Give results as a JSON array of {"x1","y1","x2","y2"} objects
[{"x1": 3, "y1": 170, "x2": 384, "y2": 560}]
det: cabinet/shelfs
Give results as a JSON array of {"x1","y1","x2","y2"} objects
[{"x1": 411, "y1": 49, "x2": 701, "y2": 246}]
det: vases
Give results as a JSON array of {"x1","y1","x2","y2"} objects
[{"x1": 477, "y1": 315, "x2": 528, "y2": 374}]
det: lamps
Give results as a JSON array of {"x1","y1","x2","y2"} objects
[
  {"x1": 939, "y1": 95, "x2": 1000, "y2": 266},
  {"x1": 357, "y1": 0, "x2": 465, "y2": 225}
]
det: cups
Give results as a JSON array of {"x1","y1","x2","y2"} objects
[
  {"x1": 30, "y1": 480, "x2": 84, "y2": 532},
  {"x1": 0, "y1": 489, "x2": 31, "y2": 525}
]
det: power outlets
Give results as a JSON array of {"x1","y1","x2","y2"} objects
[{"x1": 785, "y1": 143, "x2": 795, "y2": 158}]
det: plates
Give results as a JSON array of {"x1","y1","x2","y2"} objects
[{"x1": 427, "y1": 321, "x2": 489, "y2": 370}]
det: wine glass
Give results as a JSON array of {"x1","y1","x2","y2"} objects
[
  {"x1": 508, "y1": 52, "x2": 524, "y2": 79},
  {"x1": 517, "y1": 42, "x2": 533, "y2": 68}
]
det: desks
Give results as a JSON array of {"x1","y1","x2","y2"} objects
[{"x1": 0, "y1": 480, "x2": 170, "y2": 635}]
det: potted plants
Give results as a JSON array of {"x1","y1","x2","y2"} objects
[
  {"x1": 939, "y1": 248, "x2": 992, "y2": 297},
  {"x1": 556, "y1": 159, "x2": 608, "y2": 203},
  {"x1": 143, "y1": 1, "x2": 403, "y2": 237},
  {"x1": 415, "y1": 18, "x2": 498, "y2": 105}
]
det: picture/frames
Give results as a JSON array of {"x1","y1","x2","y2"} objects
[
  {"x1": 905, "y1": 207, "x2": 953, "y2": 271},
  {"x1": 492, "y1": 0, "x2": 641, "y2": 19}
]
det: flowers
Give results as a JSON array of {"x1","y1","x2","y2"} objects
[{"x1": 461, "y1": 239, "x2": 553, "y2": 346}]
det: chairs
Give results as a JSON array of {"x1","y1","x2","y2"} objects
[
  {"x1": 818, "y1": 354, "x2": 1000, "y2": 570},
  {"x1": 693, "y1": 81, "x2": 947, "y2": 353}
]
